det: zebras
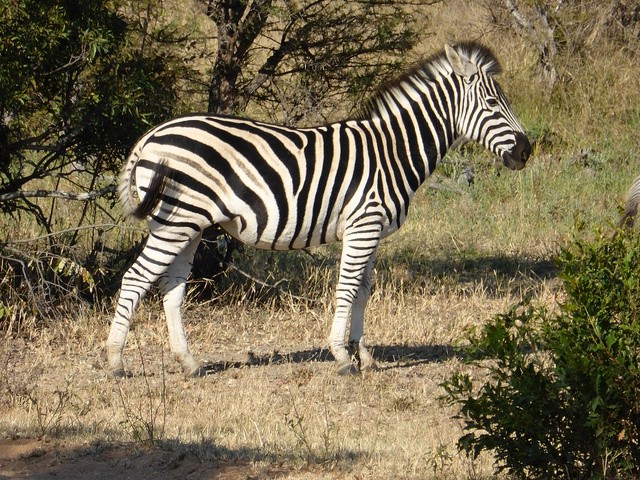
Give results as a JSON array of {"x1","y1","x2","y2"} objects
[{"x1": 106, "y1": 41, "x2": 530, "y2": 384}]
